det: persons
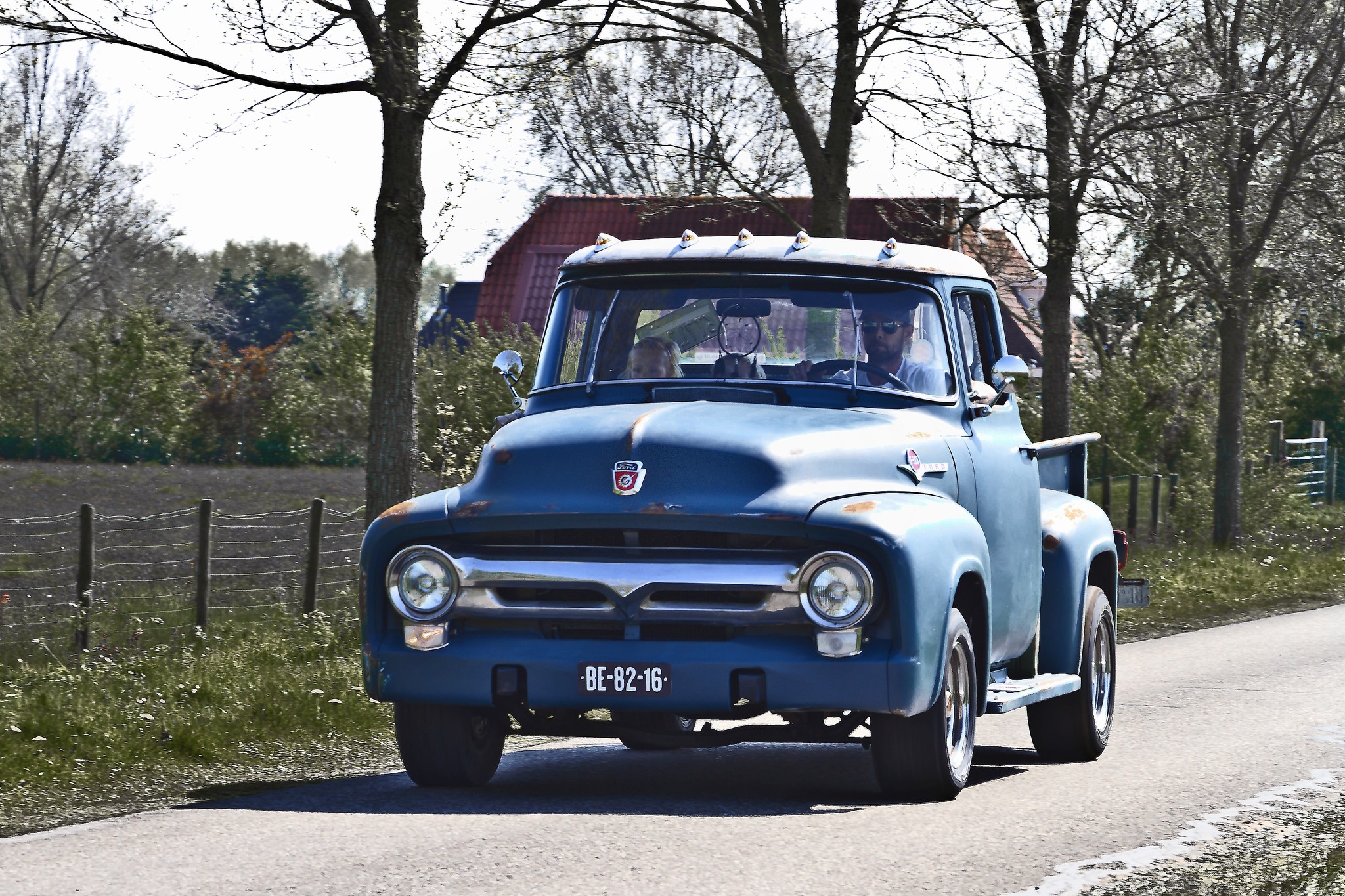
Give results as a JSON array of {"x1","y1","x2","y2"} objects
[
  {"x1": 618, "y1": 337, "x2": 684, "y2": 380},
  {"x1": 712, "y1": 353, "x2": 766, "y2": 379},
  {"x1": 789, "y1": 293, "x2": 997, "y2": 405}
]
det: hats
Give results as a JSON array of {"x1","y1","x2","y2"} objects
[{"x1": 859, "y1": 300, "x2": 915, "y2": 324}]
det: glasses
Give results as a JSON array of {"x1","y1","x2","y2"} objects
[{"x1": 860, "y1": 320, "x2": 910, "y2": 334}]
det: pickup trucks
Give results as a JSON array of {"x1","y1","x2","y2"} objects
[{"x1": 357, "y1": 227, "x2": 1149, "y2": 805}]
